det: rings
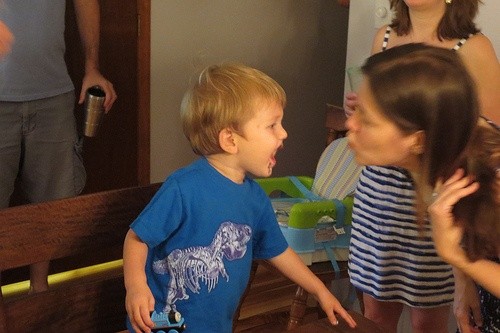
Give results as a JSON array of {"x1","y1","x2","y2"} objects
[{"x1": 430, "y1": 191, "x2": 438, "y2": 198}]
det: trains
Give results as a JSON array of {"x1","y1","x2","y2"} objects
[{"x1": 148, "y1": 309, "x2": 187, "y2": 333}]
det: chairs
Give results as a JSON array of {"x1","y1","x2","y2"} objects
[{"x1": 229, "y1": 138, "x2": 365, "y2": 333}]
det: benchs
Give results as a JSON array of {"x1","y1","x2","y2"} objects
[{"x1": 0, "y1": 182, "x2": 348, "y2": 333}]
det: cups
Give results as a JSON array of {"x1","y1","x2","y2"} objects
[
  {"x1": 347, "y1": 67, "x2": 362, "y2": 110},
  {"x1": 82, "y1": 89, "x2": 105, "y2": 137}
]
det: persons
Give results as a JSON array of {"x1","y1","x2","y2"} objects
[
  {"x1": 123, "y1": 64, "x2": 357, "y2": 333},
  {"x1": 345, "y1": 1, "x2": 500, "y2": 333},
  {"x1": 0, "y1": 0, "x2": 116, "y2": 296}
]
